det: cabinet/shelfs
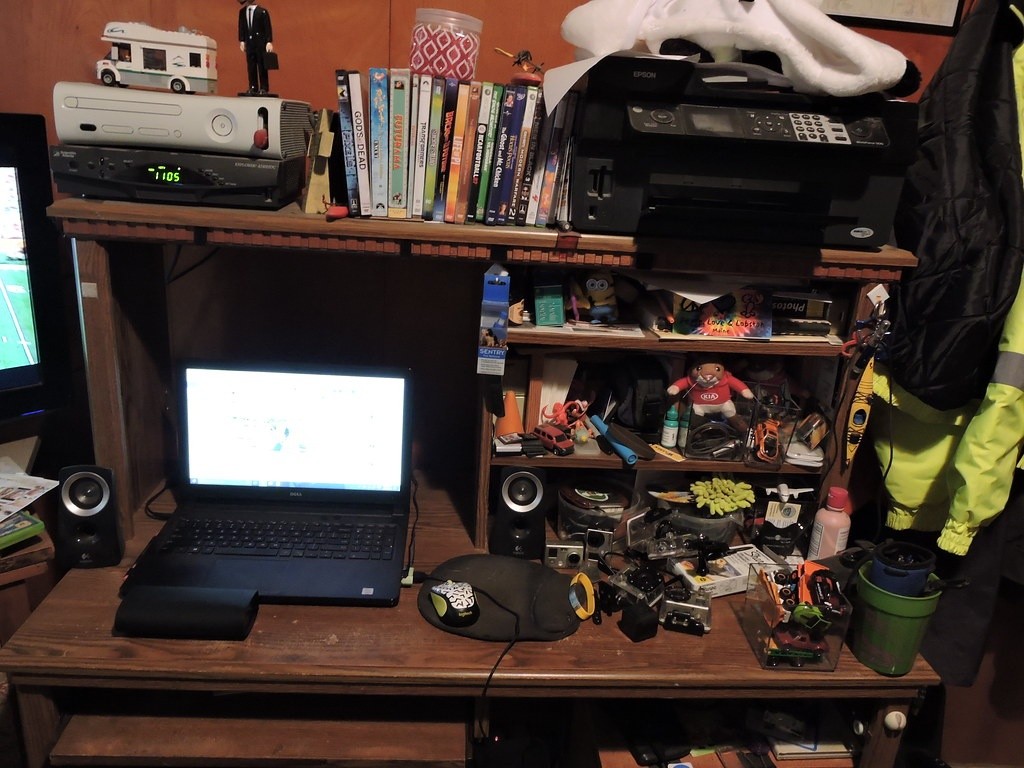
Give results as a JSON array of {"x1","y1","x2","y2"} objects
[{"x1": 0, "y1": 197, "x2": 942, "y2": 768}]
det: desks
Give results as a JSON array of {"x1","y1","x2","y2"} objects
[{"x1": 0, "y1": 508, "x2": 55, "y2": 646}]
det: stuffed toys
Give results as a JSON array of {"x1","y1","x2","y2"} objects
[{"x1": 667, "y1": 354, "x2": 752, "y2": 432}]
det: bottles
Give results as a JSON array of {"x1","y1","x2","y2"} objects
[
  {"x1": 807, "y1": 486, "x2": 852, "y2": 560},
  {"x1": 677, "y1": 405, "x2": 691, "y2": 448},
  {"x1": 408, "y1": 8, "x2": 483, "y2": 86},
  {"x1": 660, "y1": 405, "x2": 679, "y2": 447}
]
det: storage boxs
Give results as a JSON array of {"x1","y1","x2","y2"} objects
[
  {"x1": 534, "y1": 285, "x2": 563, "y2": 326},
  {"x1": 771, "y1": 292, "x2": 832, "y2": 319},
  {"x1": 639, "y1": 281, "x2": 774, "y2": 340},
  {"x1": 742, "y1": 562, "x2": 854, "y2": 671}
]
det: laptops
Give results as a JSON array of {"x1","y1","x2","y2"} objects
[{"x1": 118, "y1": 363, "x2": 416, "y2": 609}]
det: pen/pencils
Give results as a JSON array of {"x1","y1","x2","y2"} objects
[
  {"x1": 123, "y1": 530, "x2": 161, "y2": 581},
  {"x1": 656, "y1": 291, "x2": 675, "y2": 323}
]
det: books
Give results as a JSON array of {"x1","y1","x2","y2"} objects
[
  {"x1": 0, "y1": 512, "x2": 46, "y2": 552},
  {"x1": 328, "y1": 66, "x2": 578, "y2": 231}
]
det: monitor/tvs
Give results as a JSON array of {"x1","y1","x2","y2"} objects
[{"x1": 0, "y1": 113, "x2": 90, "y2": 449}]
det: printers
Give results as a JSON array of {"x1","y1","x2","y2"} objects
[{"x1": 568, "y1": 54, "x2": 922, "y2": 279}]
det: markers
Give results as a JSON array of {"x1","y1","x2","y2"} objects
[
  {"x1": 584, "y1": 414, "x2": 615, "y2": 456},
  {"x1": 591, "y1": 414, "x2": 637, "y2": 465}
]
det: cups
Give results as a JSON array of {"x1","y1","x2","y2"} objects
[{"x1": 855, "y1": 563, "x2": 942, "y2": 675}]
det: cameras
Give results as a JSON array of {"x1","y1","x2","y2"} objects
[{"x1": 544, "y1": 539, "x2": 585, "y2": 570}]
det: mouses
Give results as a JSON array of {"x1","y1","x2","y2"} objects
[{"x1": 431, "y1": 578, "x2": 480, "y2": 628}]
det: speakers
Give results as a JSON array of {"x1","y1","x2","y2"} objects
[
  {"x1": 52, "y1": 464, "x2": 125, "y2": 570},
  {"x1": 489, "y1": 466, "x2": 548, "y2": 562}
]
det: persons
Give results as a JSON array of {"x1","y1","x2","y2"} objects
[{"x1": 237, "y1": 0, "x2": 280, "y2": 93}]
